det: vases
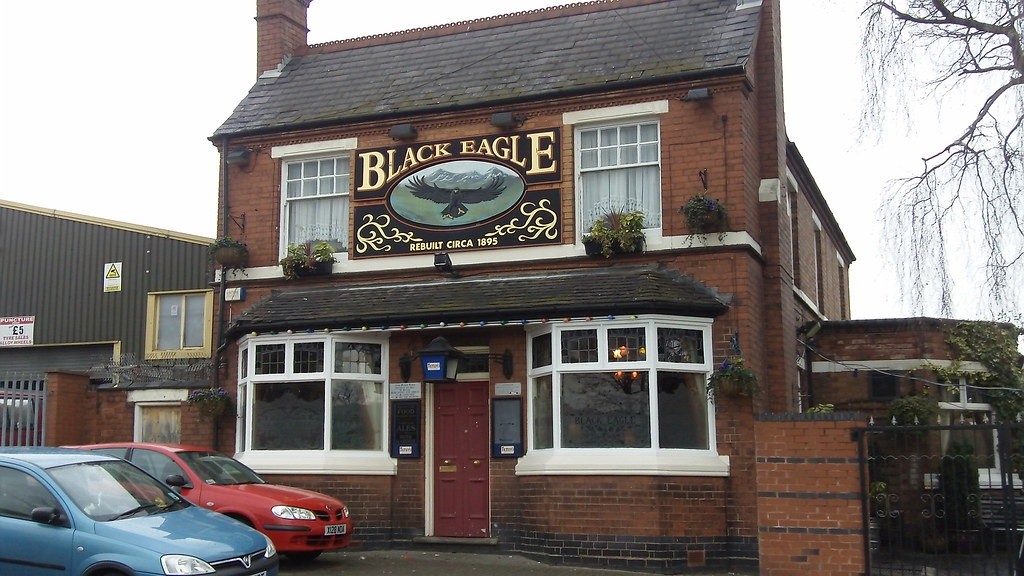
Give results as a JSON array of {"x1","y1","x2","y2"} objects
[
  {"x1": 717, "y1": 377, "x2": 746, "y2": 398},
  {"x1": 198, "y1": 398, "x2": 226, "y2": 417}
]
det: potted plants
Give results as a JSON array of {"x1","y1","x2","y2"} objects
[
  {"x1": 279, "y1": 239, "x2": 340, "y2": 281},
  {"x1": 206, "y1": 235, "x2": 250, "y2": 280},
  {"x1": 888, "y1": 395, "x2": 932, "y2": 441},
  {"x1": 581, "y1": 204, "x2": 648, "y2": 258},
  {"x1": 939, "y1": 443, "x2": 988, "y2": 553}
]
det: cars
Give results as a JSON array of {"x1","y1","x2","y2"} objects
[
  {"x1": 1, "y1": 445, "x2": 282, "y2": 576},
  {"x1": 26, "y1": 440, "x2": 354, "y2": 563}
]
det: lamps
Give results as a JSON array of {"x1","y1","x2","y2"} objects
[
  {"x1": 388, "y1": 123, "x2": 418, "y2": 141},
  {"x1": 398, "y1": 336, "x2": 513, "y2": 383},
  {"x1": 225, "y1": 149, "x2": 250, "y2": 167},
  {"x1": 682, "y1": 88, "x2": 713, "y2": 101},
  {"x1": 434, "y1": 252, "x2": 458, "y2": 277},
  {"x1": 801, "y1": 319, "x2": 824, "y2": 341},
  {"x1": 490, "y1": 112, "x2": 518, "y2": 132}
]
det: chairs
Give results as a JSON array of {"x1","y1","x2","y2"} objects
[
  {"x1": 4, "y1": 473, "x2": 36, "y2": 512},
  {"x1": 135, "y1": 458, "x2": 150, "y2": 473},
  {"x1": 161, "y1": 461, "x2": 181, "y2": 481}
]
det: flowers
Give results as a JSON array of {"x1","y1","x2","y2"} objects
[
  {"x1": 704, "y1": 353, "x2": 761, "y2": 405},
  {"x1": 186, "y1": 387, "x2": 234, "y2": 421},
  {"x1": 679, "y1": 190, "x2": 728, "y2": 248}
]
